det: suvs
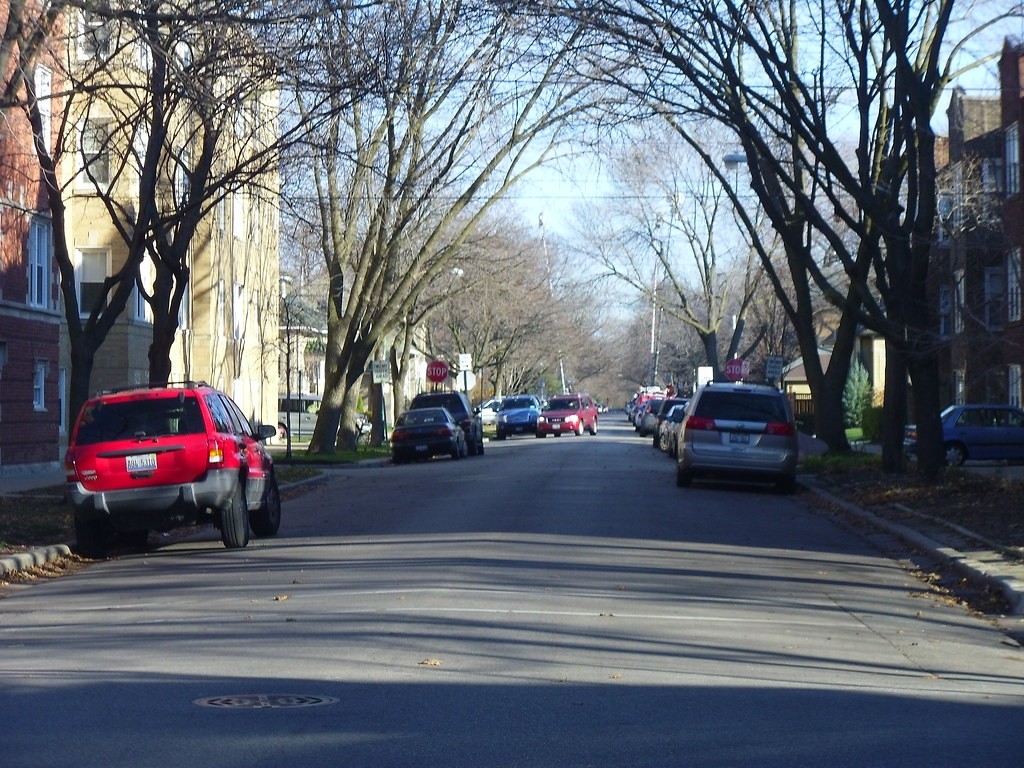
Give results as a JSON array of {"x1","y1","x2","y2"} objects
[
  {"x1": 493, "y1": 395, "x2": 545, "y2": 440},
  {"x1": 63, "y1": 377, "x2": 282, "y2": 558},
  {"x1": 535, "y1": 393, "x2": 599, "y2": 438},
  {"x1": 673, "y1": 378, "x2": 798, "y2": 495},
  {"x1": 405, "y1": 390, "x2": 486, "y2": 457}
]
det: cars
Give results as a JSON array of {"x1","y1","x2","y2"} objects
[
  {"x1": 902, "y1": 403, "x2": 1024, "y2": 467},
  {"x1": 389, "y1": 404, "x2": 468, "y2": 464},
  {"x1": 624, "y1": 391, "x2": 691, "y2": 451}
]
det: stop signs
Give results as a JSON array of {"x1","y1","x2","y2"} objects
[
  {"x1": 723, "y1": 358, "x2": 748, "y2": 381},
  {"x1": 425, "y1": 361, "x2": 448, "y2": 382}
]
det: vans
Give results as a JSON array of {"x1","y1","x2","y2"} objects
[
  {"x1": 475, "y1": 396, "x2": 503, "y2": 425},
  {"x1": 278, "y1": 393, "x2": 372, "y2": 443}
]
